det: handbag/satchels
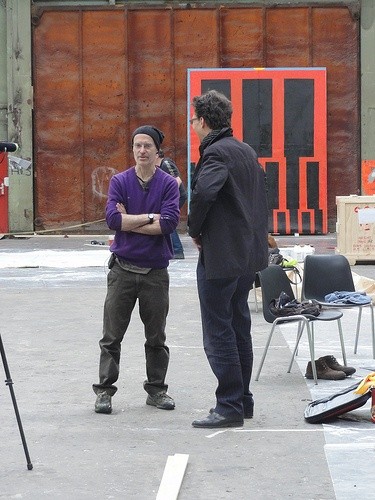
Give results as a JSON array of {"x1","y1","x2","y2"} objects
[
  {"x1": 269, "y1": 253, "x2": 303, "y2": 284},
  {"x1": 268, "y1": 291, "x2": 322, "y2": 319}
]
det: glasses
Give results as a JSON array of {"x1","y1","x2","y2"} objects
[{"x1": 188, "y1": 118, "x2": 199, "y2": 125}]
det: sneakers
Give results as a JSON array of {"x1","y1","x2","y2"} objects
[
  {"x1": 94, "y1": 391, "x2": 112, "y2": 414},
  {"x1": 146, "y1": 390, "x2": 176, "y2": 410}
]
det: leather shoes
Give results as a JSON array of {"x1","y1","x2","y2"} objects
[
  {"x1": 191, "y1": 412, "x2": 244, "y2": 428},
  {"x1": 208, "y1": 408, "x2": 253, "y2": 419}
]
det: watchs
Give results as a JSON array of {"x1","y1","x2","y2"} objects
[{"x1": 148, "y1": 213, "x2": 154, "y2": 223}]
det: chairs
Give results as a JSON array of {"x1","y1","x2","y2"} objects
[
  {"x1": 256, "y1": 266, "x2": 346, "y2": 385},
  {"x1": 296, "y1": 255, "x2": 375, "y2": 360}
]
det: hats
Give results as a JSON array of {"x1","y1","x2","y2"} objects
[{"x1": 132, "y1": 125, "x2": 165, "y2": 152}]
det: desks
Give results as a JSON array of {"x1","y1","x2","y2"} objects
[{"x1": 336, "y1": 196, "x2": 375, "y2": 266}]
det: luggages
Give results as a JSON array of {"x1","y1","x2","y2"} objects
[{"x1": 304, "y1": 373, "x2": 375, "y2": 424}]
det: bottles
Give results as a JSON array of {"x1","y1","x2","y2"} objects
[
  {"x1": 293, "y1": 243, "x2": 303, "y2": 261},
  {"x1": 303, "y1": 243, "x2": 315, "y2": 258}
]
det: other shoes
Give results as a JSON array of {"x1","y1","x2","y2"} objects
[
  {"x1": 305, "y1": 360, "x2": 346, "y2": 379},
  {"x1": 319, "y1": 355, "x2": 356, "y2": 376}
]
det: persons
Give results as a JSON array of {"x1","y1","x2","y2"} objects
[
  {"x1": 91, "y1": 125, "x2": 180, "y2": 412},
  {"x1": 187, "y1": 91, "x2": 269, "y2": 428},
  {"x1": 155, "y1": 150, "x2": 186, "y2": 258}
]
